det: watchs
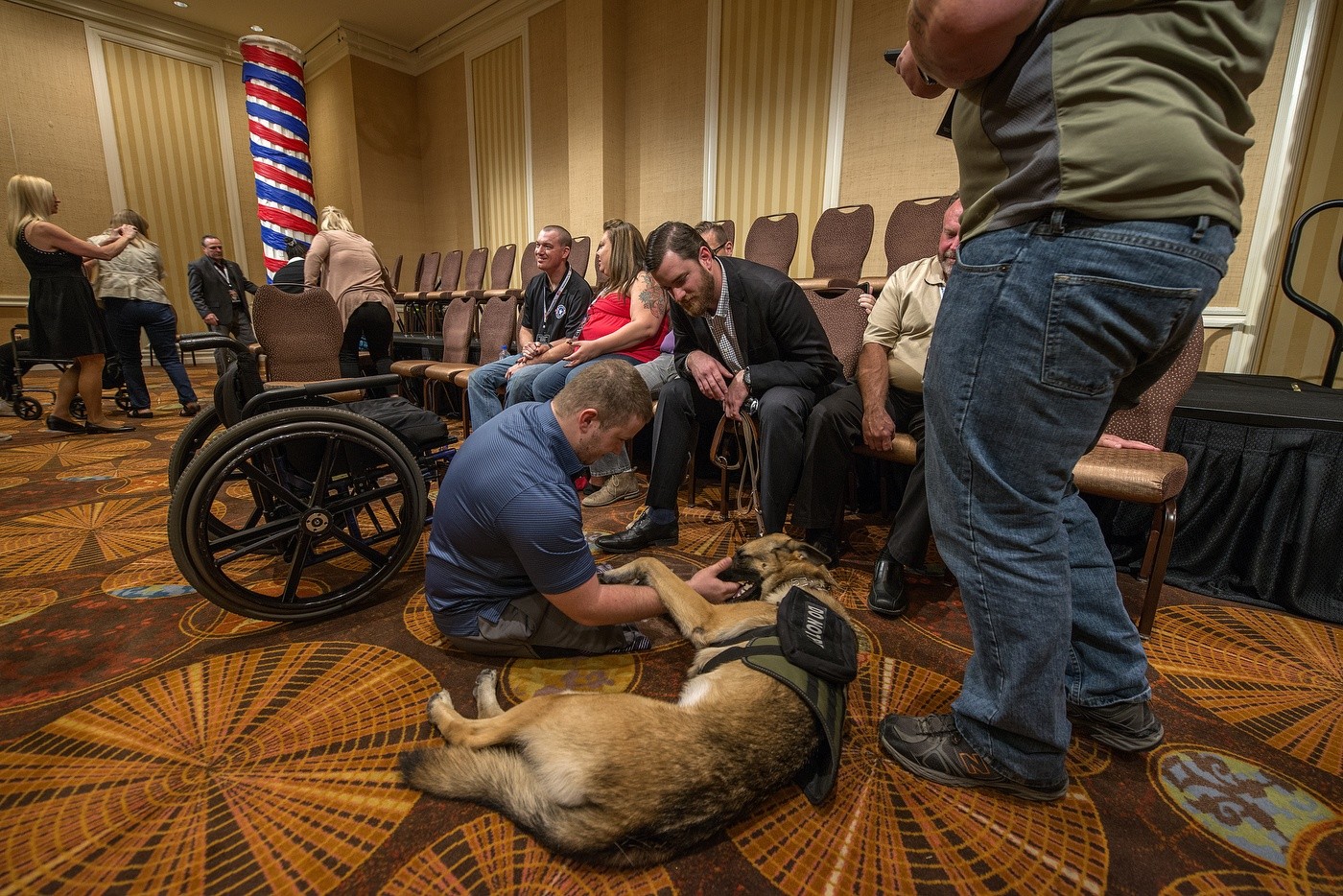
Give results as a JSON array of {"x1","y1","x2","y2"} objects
[{"x1": 743, "y1": 367, "x2": 752, "y2": 393}]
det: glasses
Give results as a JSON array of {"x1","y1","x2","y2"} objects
[{"x1": 713, "y1": 242, "x2": 727, "y2": 256}]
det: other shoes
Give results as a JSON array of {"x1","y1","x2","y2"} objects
[
  {"x1": 0, "y1": 397, "x2": 18, "y2": 416},
  {"x1": 0, "y1": 432, "x2": 13, "y2": 441}
]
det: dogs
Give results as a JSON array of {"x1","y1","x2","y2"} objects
[{"x1": 398, "y1": 534, "x2": 860, "y2": 876}]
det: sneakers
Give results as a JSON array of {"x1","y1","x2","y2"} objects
[
  {"x1": 878, "y1": 711, "x2": 1070, "y2": 802},
  {"x1": 1068, "y1": 697, "x2": 1165, "y2": 758},
  {"x1": 581, "y1": 467, "x2": 640, "y2": 507}
]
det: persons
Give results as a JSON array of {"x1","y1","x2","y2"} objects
[
  {"x1": 468, "y1": 226, "x2": 593, "y2": 431},
  {"x1": 877, "y1": 0, "x2": 1285, "y2": 800},
  {"x1": 424, "y1": 357, "x2": 738, "y2": 658},
  {"x1": 87, "y1": 210, "x2": 202, "y2": 417},
  {"x1": 595, "y1": 221, "x2": 875, "y2": 553},
  {"x1": 802, "y1": 198, "x2": 963, "y2": 621},
  {"x1": 187, "y1": 236, "x2": 259, "y2": 377},
  {"x1": 304, "y1": 206, "x2": 396, "y2": 397},
  {"x1": 5, "y1": 175, "x2": 138, "y2": 432},
  {"x1": 581, "y1": 221, "x2": 733, "y2": 531},
  {"x1": 505, "y1": 219, "x2": 670, "y2": 493},
  {"x1": 273, "y1": 237, "x2": 320, "y2": 295}
]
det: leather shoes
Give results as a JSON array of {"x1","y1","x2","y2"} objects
[
  {"x1": 46, "y1": 414, "x2": 85, "y2": 432},
  {"x1": 869, "y1": 544, "x2": 910, "y2": 614},
  {"x1": 594, "y1": 513, "x2": 680, "y2": 553},
  {"x1": 805, "y1": 530, "x2": 848, "y2": 568},
  {"x1": 86, "y1": 421, "x2": 135, "y2": 433}
]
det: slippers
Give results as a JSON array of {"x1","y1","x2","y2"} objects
[
  {"x1": 127, "y1": 409, "x2": 153, "y2": 418},
  {"x1": 179, "y1": 405, "x2": 202, "y2": 416}
]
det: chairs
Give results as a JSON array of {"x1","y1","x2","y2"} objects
[{"x1": 379, "y1": 194, "x2": 1200, "y2": 647}]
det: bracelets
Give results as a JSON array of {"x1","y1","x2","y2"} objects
[
  {"x1": 916, "y1": 66, "x2": 937, "y2": 86},
  {"x1": 547, "y1": 344, "x2": 552, "y2": 348}
]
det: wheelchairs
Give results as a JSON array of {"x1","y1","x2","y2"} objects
[
  {"x1": 166, "y1": 332, "x2": 461, "y2": 625},
  {"x1": 0, "y1": 323, "x2": 140, "y2": 422}
]
